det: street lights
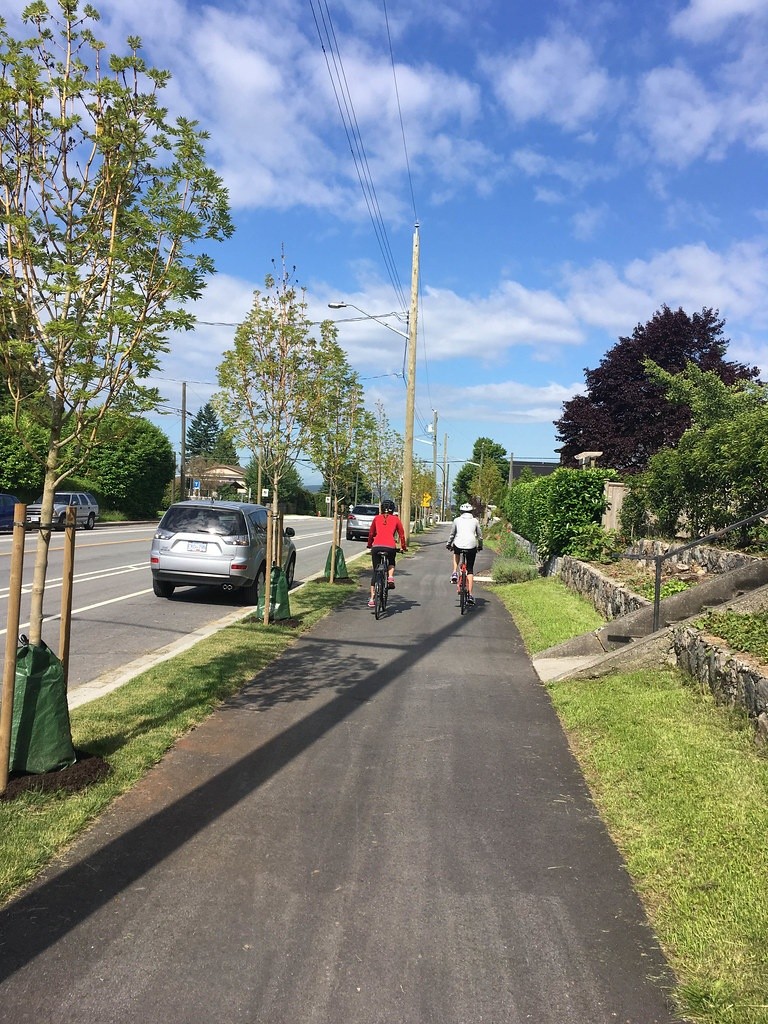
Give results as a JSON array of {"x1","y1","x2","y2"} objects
[{"x1": 328, "y1": 302, "x2": 417, "y2": 549}]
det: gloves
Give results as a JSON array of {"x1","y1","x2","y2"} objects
[
  {"x1": 478, "y1": 546, "x2": 482, "y2": 551},
  {"x1": 447, "y1": 545, "x2": 451, "y2": 550}
]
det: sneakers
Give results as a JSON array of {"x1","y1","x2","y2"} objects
[
  {"x1": 449, "y1": 573, "x2": 457, "y2": 582},
  {"x1": 468, "y1": 595, "x2": 476, "y2": 605},
  {"x1": 387, "y1": 578, "x2": 395, "y2": 589},
  {"x1": 368, "y1": 601, "x2": 376, "y2": 607}
]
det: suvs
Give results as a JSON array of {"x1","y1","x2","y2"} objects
[{"x1": 26, "y1": 491, "x2": 98, "y2": 532}]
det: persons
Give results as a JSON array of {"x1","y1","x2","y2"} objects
[
  {"x1": 368, "y1": 500, "x2": 408, "y2": 607},
  {"x1": 446, "y1": 502, "x2": 484, "y2": 604}
]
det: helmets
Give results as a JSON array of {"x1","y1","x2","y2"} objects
[
  {"x1": 460, "y1": 503, "x2": 473, "y2": 511},
  {"x1": 381, "y1": 500, "x2": 395, "y2": 511}
]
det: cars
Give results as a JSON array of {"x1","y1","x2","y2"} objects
[
  {"x1": 0, "y1": 494, "x2": 21, "y2": 531},
  {"x1": 346, "y1": 505, "x2": 380, "y2": 541},
  {"x1": 150, "y1": 500, "x2": 296, "y2": 605}
]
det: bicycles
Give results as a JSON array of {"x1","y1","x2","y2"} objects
[
  {"x1": 446, "y1": 545, "x2": 483, "y2": 616},
  {"x1": 366, "y1": 545, "x2": 409, "y2": 620}
]
